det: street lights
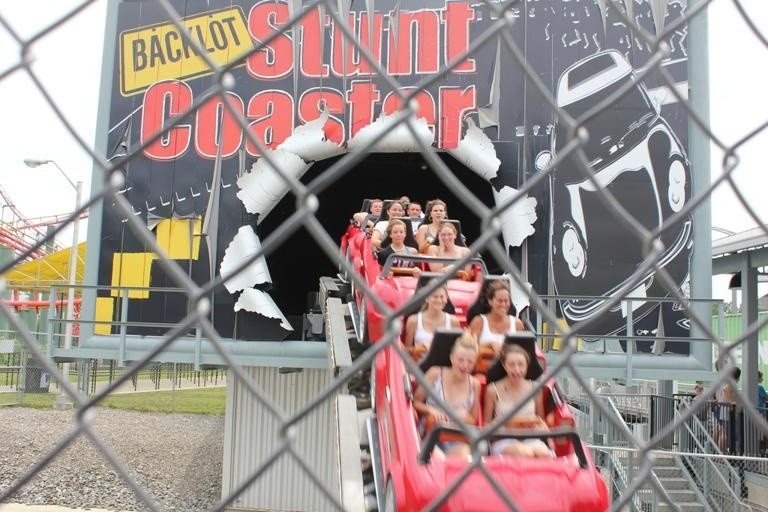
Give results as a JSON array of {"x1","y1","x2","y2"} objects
[{"x1": 23, "y1": 156, "x2": 83, "y2": 409}]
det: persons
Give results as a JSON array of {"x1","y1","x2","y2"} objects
[
  {"x1": 705, "y1": 393, "x2": 718, "y2": 443},
  {"x1": 691, "y1": 383, "x2": 711, "y2": 445},
  {"x1": 714, "y1": 359, "x2": 735, "y2": 450},
  {"x1": 720, "y1": 366, "x2": 742, "y2": 456},
  {"x1": 757, "y1": 371, "x2": 768, "y2": 452},
  {"x1": 343, "y1": 192, "x2": 558, "y2": 459}
]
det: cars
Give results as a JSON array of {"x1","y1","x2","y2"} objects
[{"x1": 532, "y1": 48, "x2": 692, "y2": 346}]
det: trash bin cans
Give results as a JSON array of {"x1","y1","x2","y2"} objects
[{"x1": 25, "y1": 358, "x2": 51, "y2": 393}]
